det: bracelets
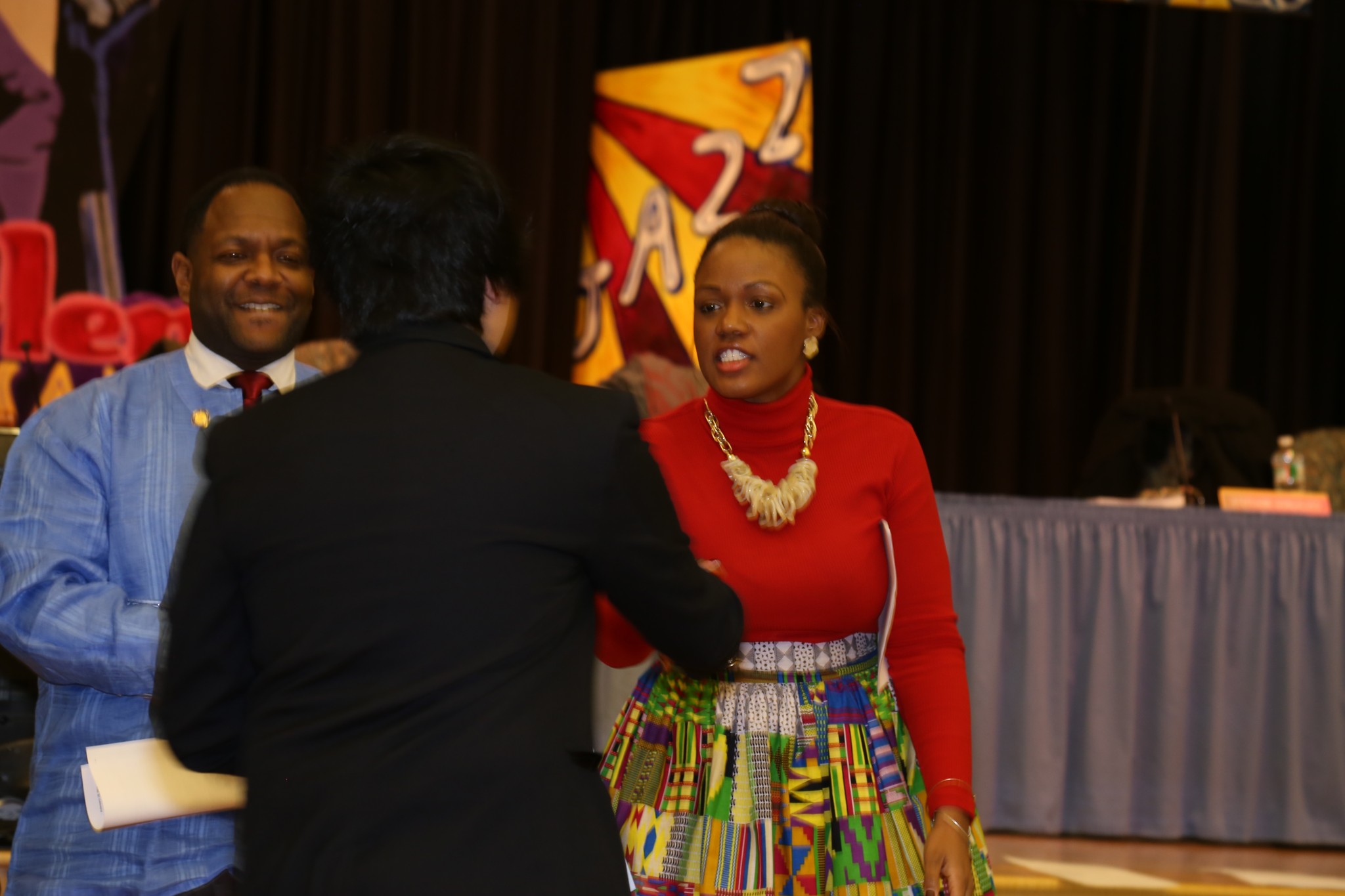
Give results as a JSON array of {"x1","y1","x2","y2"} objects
[
  {"x1": 931, "y1": 808, "x2": 973, "y2": 855},
  {"x1": 925, "y1": 778, "x2": 976, "y2": 819}
]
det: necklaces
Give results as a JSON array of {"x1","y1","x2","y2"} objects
[{"x1": 704, "y1": 390, "x2": 819, "y2": 530}]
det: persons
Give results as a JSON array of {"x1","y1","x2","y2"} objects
[
  {"x1": 595, "y1": 196, "x2": 997, "y2": 896},
  {"x1": 0, "y1": 168, "x2": 361, "y2": 896},
  {"x1": 147, "y1": 134, "x2": 744, "y2": 896}
]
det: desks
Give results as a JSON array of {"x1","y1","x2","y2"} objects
[{"x1": 932, "y1": 492, "x2": 1345, "y2": 848}]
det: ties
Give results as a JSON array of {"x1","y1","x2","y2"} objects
[{"x1": 227, "y1": 371, "x2": 274, "y2": 410}]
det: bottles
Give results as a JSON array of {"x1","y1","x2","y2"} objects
[{"x1": 1273, "y1": 435, "x2": 1307, "y2": 490}]
